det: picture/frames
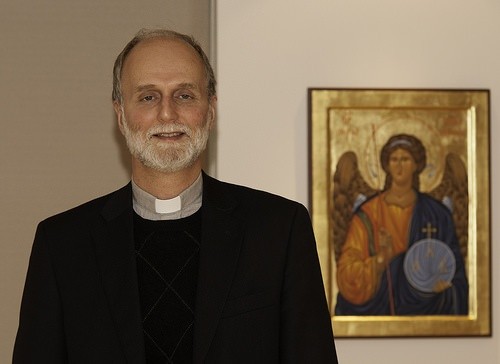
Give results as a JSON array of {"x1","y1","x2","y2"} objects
[{"x1": 307, "y1": 86, "x2": 495, "y2": 339}]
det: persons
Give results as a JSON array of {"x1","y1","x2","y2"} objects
[{"x1": 12, "y1": 26, "x2": 340, "y2": 364}]
